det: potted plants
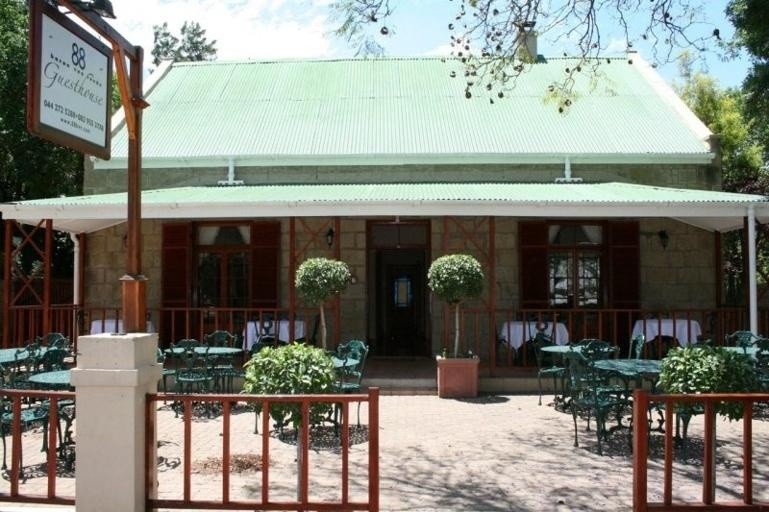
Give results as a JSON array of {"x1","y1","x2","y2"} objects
[{"x1": 426, "y1": 252, "x2": 487, "y2": 399}]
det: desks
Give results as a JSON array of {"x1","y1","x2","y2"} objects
[
  {"x1": 501, "y1": 320, "x2": 564, "y2": 369},
  {"x1": 635, "y1": 317, "x2": 698, "y2": 359},
  {"x1": 247, "y1": 319, "x2": 304, "y2": 351}
]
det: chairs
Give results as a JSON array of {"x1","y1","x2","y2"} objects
[{"x1": 529, "y1": 329, "x2": 769, "y2": 457}]
